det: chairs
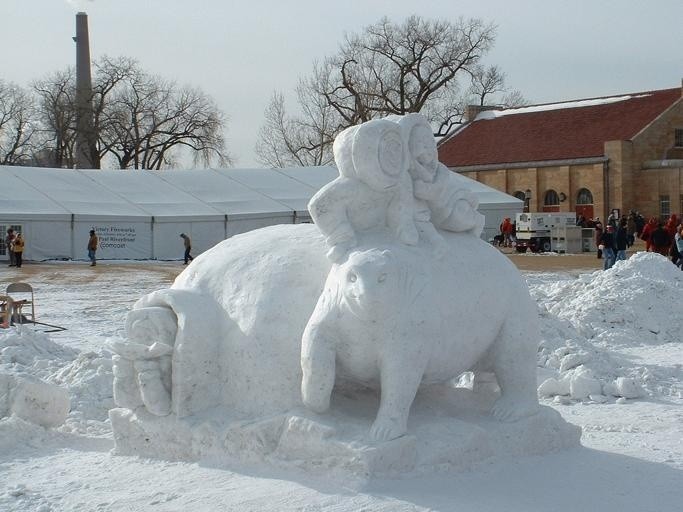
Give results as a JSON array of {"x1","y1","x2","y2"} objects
[{"x1": 5, "y1": 281, "x2": 34, "y2": 328}]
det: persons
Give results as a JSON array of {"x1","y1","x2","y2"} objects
[
  {"x1": 510, "y1": 219, "x2": 516, "y2": 244},
  {"x1": 4, "y1": 228, "x2": 16, "y2": 267},
  {"x1": 500, "y1": 217, "x2": 511, "y2": 248},
  {"x1": 179, "y1": 233, "x2": 193, "y2": 265},
  {"x1": 86, "y1": 229, "x2": 98, "y2": 267},
  {"x1": 9, "y1": 232, "x2": 25, "y2": 268},
  {"x1": 575, "y1": 207, "x2": 682, "y2": 272},
  {"x1": 498, "y1": 217, "x2": 511, "y2": 246}
]
